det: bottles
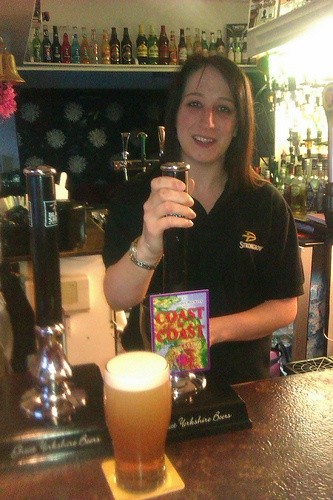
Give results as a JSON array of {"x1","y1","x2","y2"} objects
[
  {"x1": 260, "y1": 129, "x2": 328, "y2": 221},
  {"x1": 31, "y1": 23, "x2": 242, "y2": 65}
]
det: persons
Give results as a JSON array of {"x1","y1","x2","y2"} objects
[{"x1": 102, "y1": 53, "x2": 304, "y2": 386}]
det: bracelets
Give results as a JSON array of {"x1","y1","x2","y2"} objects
[{"x1": 128, "y1": 240, "x2": 157, "y2": 270}]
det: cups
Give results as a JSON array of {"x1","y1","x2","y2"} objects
[{"x1": 101, "y1": 350, "x2": 173, "y2": 493}]
